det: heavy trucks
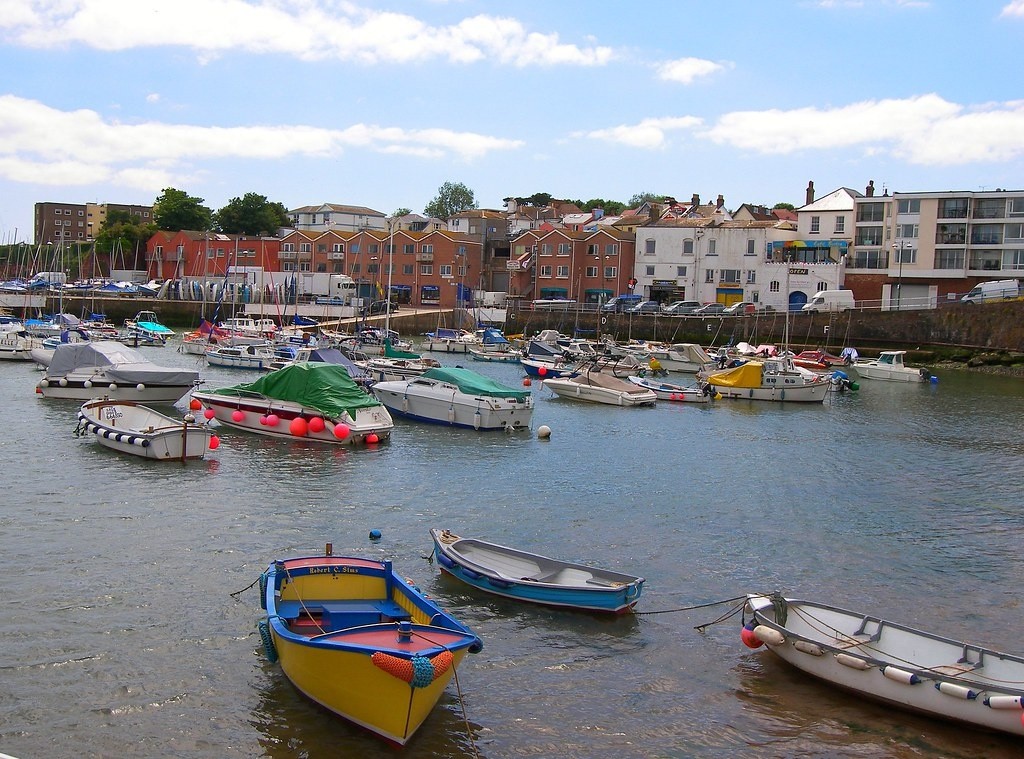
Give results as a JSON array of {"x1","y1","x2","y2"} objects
[{"x1": 254, "y1": 270, "x2": 357, "y2": 304}]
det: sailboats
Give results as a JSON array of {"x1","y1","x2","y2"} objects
[{"x1": 0, "y1": 218, "x2": 855, "y2": 379}]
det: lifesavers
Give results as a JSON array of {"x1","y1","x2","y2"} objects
[
  {"x1": 259, "y1": 621, "x2": 278, "y2": 663},
  {"x1": 259, "y1": 572, "x2": 267, "y2": 608}
]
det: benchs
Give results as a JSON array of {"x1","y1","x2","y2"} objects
[
  {"x1": 277, "y1": 598, "x2": 413, "y2": 624},
  {"x1": 586, "y1": 577, "x2": 626, "y2": 588},
  {"x1": 823, "y1": 632, "x2": 879, "y2": 648},
  {"x1": 927, "y1": 658, "x2": 983, "y2": 676},
  {"x1": 526, "y1": 571, "x2": 558, "y2": 581}
]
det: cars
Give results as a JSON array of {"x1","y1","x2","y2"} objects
[
  {"x1": 722, "y1": 303, "x2": 755, "y2": 317},
  {"x1": 360, "y1": 300, "x2": 399, "y2": 316},
  {"x1": 625, "y1": 301, "x2": 660, "y2": 315},
  {"x1": 600, "y1": 297, "x2": 641, "y2": 314},
  {"x1": 690, "y1": 303, "x2": 727, "y2": 317},
  {"x1": 661, "y1": 300, "x2": 701, "y2": 316}
]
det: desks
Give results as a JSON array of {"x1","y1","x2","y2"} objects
[{"x1": 321, "y1": 604, "x2": 381, "y2": 634}]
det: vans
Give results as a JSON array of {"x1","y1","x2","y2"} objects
[
  {"x1": 960, "y1": 279, "x2": 1021, "y2": 305},
  {"x1": 801, "y1": 290, "x2": 855, "y2": 313}
]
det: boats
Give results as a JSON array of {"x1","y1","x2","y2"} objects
[
  {"x1": 542, "y1": 354, "x2": 658, "y2": 406},
  {"x1": 189, "y1": 359, "x2": 395, "y2": 445},
  {"x1": 428, "y1": 528, "x2": 645, "y2": 615},
  {"x1": 267, "y1": 347, "x2": 377, "y2": 393},
  {"x1": 852, "y1": 350, "x2": 931, "y2": 383},
  {"x1": 627, "y1": 375, "x2": 718, "y2": 404},
  {"x1": 745, "y1": 592, "x2": 1023, "y2": 736},
  {"x1": 267, "y1": 542, "x2": 486, "y2": 747},
  {"x1": 77, "y1": 393, "x2": 219, "y2": 466},
  {"x1": 123, "y1": 309, "x2": 176, "y2": 342},
  {"x1": 34, "y1": 343, "x2": 205, "y2": 406},
  {"x1": 370, "y1": 364, "x2": 535, "y2": 431}
]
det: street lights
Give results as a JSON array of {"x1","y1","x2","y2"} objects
[{"x1": 891, "y1": 240, "x2": 913, "y2": 310}]
